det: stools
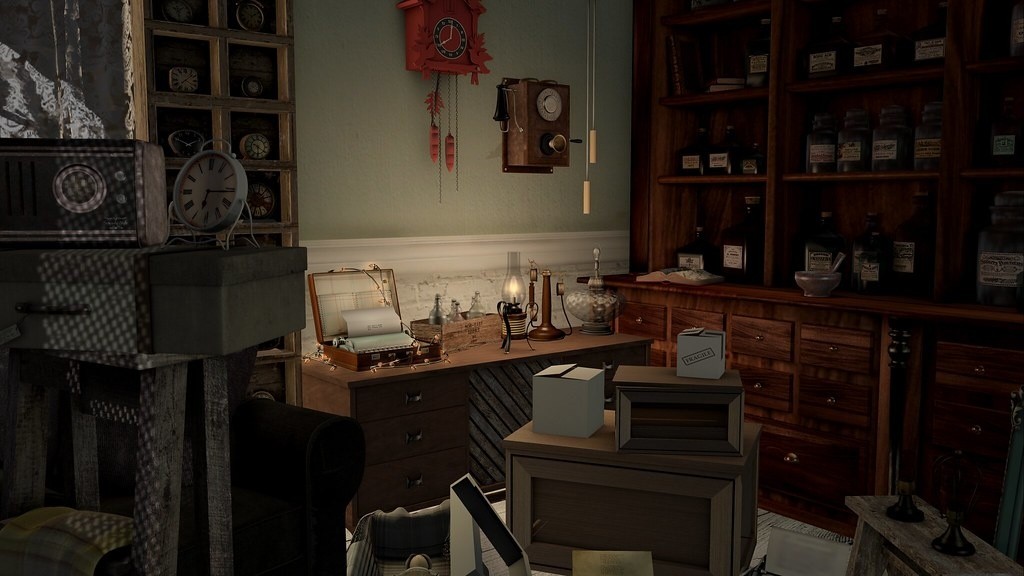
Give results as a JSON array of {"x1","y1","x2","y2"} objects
[{"x1": 0, "y1": 506, "x2": 134, "y2": 576}]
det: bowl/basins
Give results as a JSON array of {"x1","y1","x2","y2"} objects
[{"x1": 795, "y1": 271, "x2": 842, "y2": 297}]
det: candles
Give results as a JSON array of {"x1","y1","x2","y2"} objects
[{"x1": 496, "y1": 250, "x2": 538, "y2": 356}]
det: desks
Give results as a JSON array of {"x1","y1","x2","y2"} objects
[
  {"x1": 503, "y1": 408, "x2": 764, "y2": 575},
  {"x1": 845, "y1": 490, "x2": 1024, "y2": 576}
]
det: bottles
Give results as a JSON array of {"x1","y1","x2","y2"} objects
[
  {"x1": 719, "y1": 197, "x2": 765, "y2": 283},
  {"x1": 914, "y1": 2, "x2": 947, "y2": 68},
  {"x1": 678, "y1": 227, "x2": 714, "y2": 271},
  {"x1": 467, "y1": 291, "x2": 486, "y2": 318},
  {"x1": 990, "y1": 98, "x2": 1018, "y2": 167},
  {"x1": 743, "y1": 143, "x2": 763, "y2": 175},
  {"x1": 429, "y1": 293, "x2": 447, "y2": 324},
  {"x1": 709, "y1": 126, "x2": 741, "y2": 175},
  {"x1": 676, "y1": 128, "x2": 710, "y2": 175},
  {"x1": 449, "y1": 300, "x2": 466, "y2": 323},
  {"x1": 802, "y1": 103, "x2": 942, "y2": 173},
  {"x1": 805, "y1": 212, "x2": 849, "y2": 291},
  {"x1": 893, "y1": 192, "x2": 933, "y2": 300},
  {"x1": 807, "y1": 17, "x2": 852, "y2": 80},
  {"x1": 851, "y1": 212, "x2": 890, "y2": 296},
  {"x1": 977, "y1": 190, "x2": 1024, "y2": 311},
  {"x1": 853, "y1": 9, "x2": 905, "y2": 72},
  {"x1": 747, "y1": 19, "x2": 771, "y2": 88}
]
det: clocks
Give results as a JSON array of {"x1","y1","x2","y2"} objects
[
  {"x1": 161, "y1": 0, "x2": 193, "y2": 22},
  {"x1": 168, "y1": 129, "x2": 204, "y2": 158},
  {"x1": 396, "y1": 0, "x2": 492, "y2": 203},
  {"x1": 240, "y1": 134, "x2": 271, "y2": 160},
  {"x1": 241, "y1": 75, "x2": 263, "y2": 97},
  {"x1": 170, "y1": 63, "x2": 199, "y2": 93},
  {"x1": 242, "y1": 183, "x2": 275, "y2": 220},
  {"x1": 236, "y1": 0, "x2": 264, "y2": 32},
  {"x1": 160, "y1": 137, "x2": 262, "y2": 250}
]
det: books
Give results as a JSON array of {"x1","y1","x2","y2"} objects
[{"x1": 638, "y1": 265, "x2": 727, "y2": 286}]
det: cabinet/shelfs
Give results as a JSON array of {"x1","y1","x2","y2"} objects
[
  {"x1": 301, "y1": 326, "x2": 656, "y2": 537},
  {"x1": 570, "y1": 0, "x2": 1024, "y2": 568}
]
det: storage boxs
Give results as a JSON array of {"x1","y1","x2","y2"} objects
[
  {"x1": 411, "y1": 310, "x2": 501, "y2": 352},
  {"x1": 677, "y1": 328, "x2": 726, "y2": 379},
  {"x1": 533, "y1": 363, "x2": 604, "y2": 438},
  {"x1": 0, "y1": 135, "x2": 307, "y2": 356}
]
